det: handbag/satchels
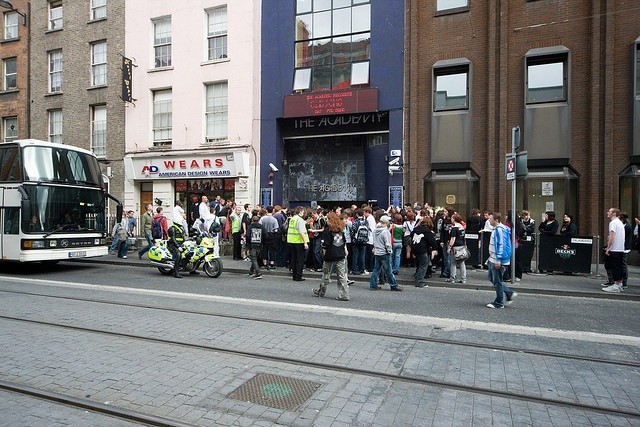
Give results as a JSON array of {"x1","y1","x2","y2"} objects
[{"x1": 453, "y1": 226, "x2": 472, "y2": 263}]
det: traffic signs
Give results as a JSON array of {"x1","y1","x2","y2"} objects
[
  {"x1": 515, "y1": 127, "x2": 520, "y2": 149},
  {"x1": 505, "y1": 157, "x2": 515, "y2": 180},
  {"x1": 516, "y1": 150, "x2": 527, "y2": 179}
]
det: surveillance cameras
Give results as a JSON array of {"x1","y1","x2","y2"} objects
[
  {"x1": 389, "y1": 156, "x2": 400, "y2": 165},
  {"x1": 269, "y1": 162, "x2": 280, "y2": 172}
]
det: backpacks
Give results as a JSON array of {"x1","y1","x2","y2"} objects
[
  {"x1": 153, "y1": 217, "x2": 164, "y2": 240},
  {"x1": 355, "y1": 220, "x2": 370, "y2": 244}
]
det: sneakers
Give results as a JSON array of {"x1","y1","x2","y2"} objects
[
  {"x1": 251, "y1": 275, "x2": 263, "y2": 279},
  {"x1": 488, "y1": 303, "x2": 505, "y2": 309},
  {"x1": 600, "y1": 282, "x2": 627, "y2": 293}
]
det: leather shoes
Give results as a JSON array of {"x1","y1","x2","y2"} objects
[{"x1": 174, "y1": 272, "x2": 183, "y2": 279}]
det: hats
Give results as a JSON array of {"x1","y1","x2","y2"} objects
[{"x1": 380, "y1": 216, "x2": 392, "y2": 222}]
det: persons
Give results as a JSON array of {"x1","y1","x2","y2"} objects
[
  {"x1": 245, "y1": 216, "x2": 268, "y2": 278},
  {"x1": 331, "y1": 213, "x2": 356, "y2": 285},
  {"x1": 28, "y1": 214, "x2": 41, "y2": 231},
  {"x1": 287, "y1": 206, "x2": 309, "y2": 281},
  {"x1": 272, "y1": 206, "x2": 285, "y2": 267},
  {"x1": 479, "y1": 211, "x2": 492, "y2": 272},
  {"x1": 602, "y1": 207, "x2": 624, "y2": 293},
  {"x1": 521, "y1": 210, "x2": 535, "y2": 274},
  {"x1": 409, "y1": 211, "x2": 438, "y2": 289},
  {"x1": 601, "y1": 212, "x2": 631, "y2": 289},
  {"x1": 389, "y1": 214, "x2": 403, "y2": 275},
  {"x1": 486, "y1": 212, "x2": 518, "y2": 309},
  {"x1": 221, "y1": 199, "x2": 232, "y2": 245},
  {"x1": 204, "y1": 207, "x2": 220, "y2": 257},
  {"x1": 255, "y1": 204, "x2": 262, "y2": 210},
  {"x1": 445, "y1": 214, "x2": 467, "y2": 283},
  {"x1": 403, "y1": 212, "x2": 416, "y2": 268},
  {"x1": 243, "y1": 203, "x2": 254, "y2": 261},
  {"x1": 538, "y1": 210, "x2": 558, "y2": 275},
  {"x1": 375, "y1": 201, "x2": 432, "y2": 214},
  {"x1": 167, "y1": 215, "x2": 200, "y2": 277},
  {"x1": 363, "y1": 207, "x2": 375, "y2": 274},
  {"x1": 109, "y1": 209, "x2": 132, "y2": 259},
  {"x1": 555, "y1": 213, "x2": 577, "y2": 247},
  {"x1": 258, "y1": 205, "x2": 279, "y2": 268},
  {"x1": 221, "y1": 197, "x2": 226, "y2": 208},
  {"x1": 440, "y1": 209, "x2": 467, "y2": 278},
  {"x1": 230, "y1": 206, "x2": 243, "y2": 261},
  {"x1": 309, "y1": 210, "x2": 325, "y2": 272},
  {"x1": 171, "y1": 200, "x2": 189, "y2": 236},
  {"x1": 117, "y1": 211, "x2": 137, "y2": 257},
  {"x1": 151, "y1": 207, "x2": 168, "y2": 239},
  {"x1": 210, "y1": 195, "x2": 221, "y2": 210},
  {"x1": 432, "y1": 210, "x2": 447, "y2": 274},
  {"x1": 633, "y1": 216, "x2": 640, "y2": 253},
  {"x1": 370, "y1": 214, "x2": 405, "y2": 291},
  {"x1": 354, "y1": 210, "x2": 372, "y2": 275},
  {"x1": 309, "y1": 202, "x2": 367, "y2": 212},
  {"x1": 468, "y1": 207, "x2": 482, "y2": 230},
  {"x1": 312, "y1": 211, "x2": 351, "y2": 302},
  {"x1": 507, "y1": 209, "x2": 521, "y2": 224},
  {"x1": 138, "y1": 203, "x2": 154, "y2": 260},
  {"x1": 188, "y1": 196, "x2": 200, "y2": 224},
  {"x1": 198, "y1": 196, "x2": 211, "y2": 219}
]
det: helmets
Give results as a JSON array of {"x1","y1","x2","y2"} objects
[{"x1": 173, "y1": 216, "x2": 184, "y2": 226}]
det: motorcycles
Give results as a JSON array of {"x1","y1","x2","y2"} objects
[{"x1": 148, "y1": 216, "x2": 223, "y2": 278}]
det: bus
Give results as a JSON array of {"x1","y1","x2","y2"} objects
[{"x1": 0, "y1": 139, "x2": 123, "y2": 265}]
